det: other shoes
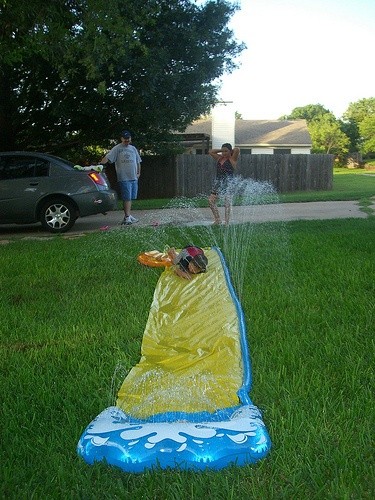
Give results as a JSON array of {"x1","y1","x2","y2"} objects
[
  {"x1": 132, "y1": 216, "x2": 139, "y2": 223},
  {"x1": 124, "y1": 217, "x2": 132, "y2": 224}
]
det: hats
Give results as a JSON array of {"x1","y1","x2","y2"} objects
[{"x1": 121, "y1": 131, "x2": 131, "y2": 138}]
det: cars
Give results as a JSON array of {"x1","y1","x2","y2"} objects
[{"x1": 1, "y1": 150, "x2": 120, "y2": 234}]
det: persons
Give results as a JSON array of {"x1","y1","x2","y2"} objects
[
  {"x1": 207, "y1": 143, "x2": 241, "y2": 228},
  {"x1": 166, "y1": 245, "x2": 208, "y2": 281},
  {"x1": 97, "y1": 131, "x2": 142, "y2": 226}
]
